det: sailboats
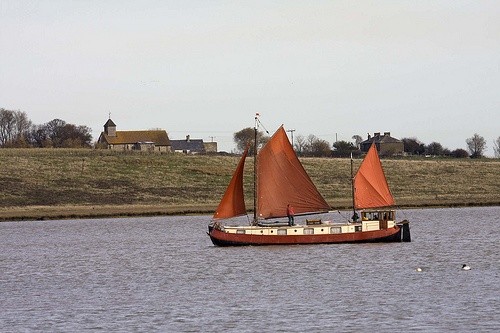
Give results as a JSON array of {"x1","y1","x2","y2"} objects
[{"x1": 205, "y1": 122, "x2": 411, "y2": 247}]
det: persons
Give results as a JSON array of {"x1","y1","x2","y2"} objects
[
  {"x1": 287, "y1": 204, "x2": 296, "y2": 226},
  {"x1": 352, "y1": 213, "x2": 391, "y2": 223}
]
